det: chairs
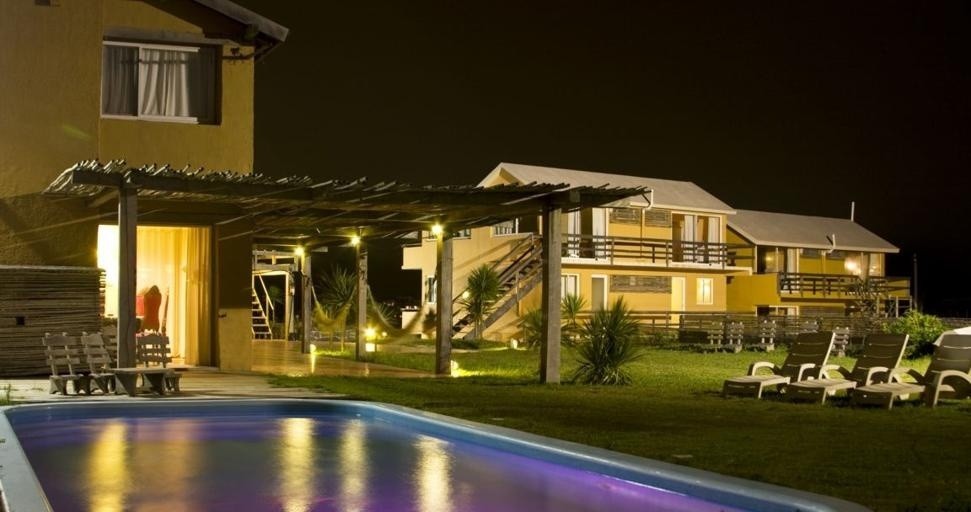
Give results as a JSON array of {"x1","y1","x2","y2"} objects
[
  {"x1": 707, "y1": 320, "x2": 971, "y2": 410},
  {"x1": 42, "y1": 324, "x2": 182, "y2": 397}
]
